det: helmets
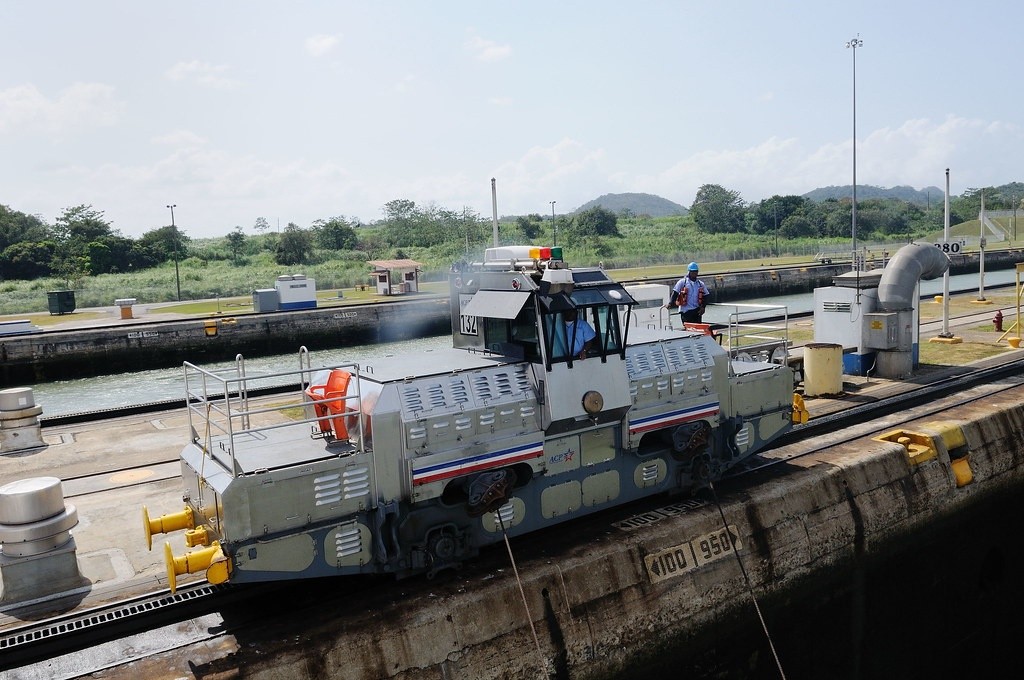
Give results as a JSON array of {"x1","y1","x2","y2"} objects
[{"x1": 687, "y1": 262, "x2": 699, "y2": 271}]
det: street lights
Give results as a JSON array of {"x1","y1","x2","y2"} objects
[
  {"x1": 845, "y1": 36, "x2": 864, "y2": 270},
  {"x1": 548, "y1": 200, "x2": 557, "y2": 248},
  {"x1": 167, "y1": 204, "x2": 182, "y2": 302}
]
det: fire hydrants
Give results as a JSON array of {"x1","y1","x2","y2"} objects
[{"x1": 993, "y1": 309, "x2": 1004, "y2": 332}]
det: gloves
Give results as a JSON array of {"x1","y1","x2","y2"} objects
[
  {"x1": 666, "y1": 304, "x2": 676, "y2": 309},
  {"x1": 698, "y1": 308, "x2": 705, "y2": 316}
]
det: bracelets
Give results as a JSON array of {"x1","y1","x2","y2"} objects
[{"x1": 582, "y1": 349, "x2": 587, "y2": 352}]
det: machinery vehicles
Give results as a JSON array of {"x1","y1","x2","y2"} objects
[{"x1": 143, "y1": 241, "x2": 795, "y2": 609}]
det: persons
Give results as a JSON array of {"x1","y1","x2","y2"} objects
[
  {"x1": 546, "y1": 308, "x2": 596, "y2": 361},
  {"x1": 661, "y1": 262, "x2": 710, "y2": 326}
]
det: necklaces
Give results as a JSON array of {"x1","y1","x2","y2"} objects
[{"x1": 565, "y1": 321, "x2": 575, "y2": 328}]
